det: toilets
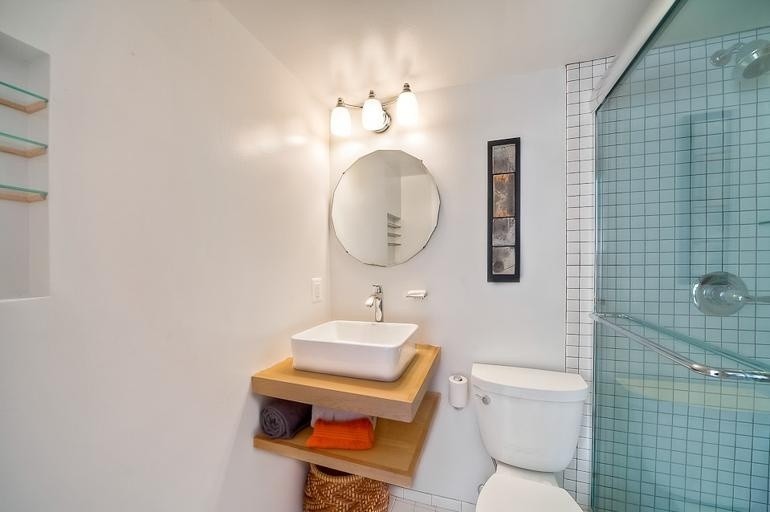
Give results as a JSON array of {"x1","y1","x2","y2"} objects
[
  {"x1": 617, "y1": 377, "x2": 769, "y2": 512},
  {"x1": 469, "y1": 364, "x2": 589, "y2": 512}
]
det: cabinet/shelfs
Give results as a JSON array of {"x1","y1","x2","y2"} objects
[{"x1": 250, "y1": 344, "x2": 442, "y2": 489}]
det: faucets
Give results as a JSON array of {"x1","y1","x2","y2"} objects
[{"x1": 365, "y1": 283, "x2": 383, "y2": 323}]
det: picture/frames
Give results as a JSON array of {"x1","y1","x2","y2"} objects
[
  {"x1": 680, "y1": 108, "x2": 729, "y2": 290},
  {"x1": 486, "y1": 137, "x2": 520, "y2": 283}
]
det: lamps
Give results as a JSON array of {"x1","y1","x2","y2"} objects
[{"x1": 330, "y1": 82, "x2": 422, "y2": 138}]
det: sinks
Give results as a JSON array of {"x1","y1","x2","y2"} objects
[{"x1": 288, "y1": 320, "x2": 420, "y2": 382}]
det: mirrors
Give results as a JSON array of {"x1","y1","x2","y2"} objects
[{"x1": 330, "y1": 149, "x2": 441, "y2": 269}]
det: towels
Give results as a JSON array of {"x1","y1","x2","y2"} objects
[{"x1": 258, "y1": 403, "x2": 377, "y2": 450}]
